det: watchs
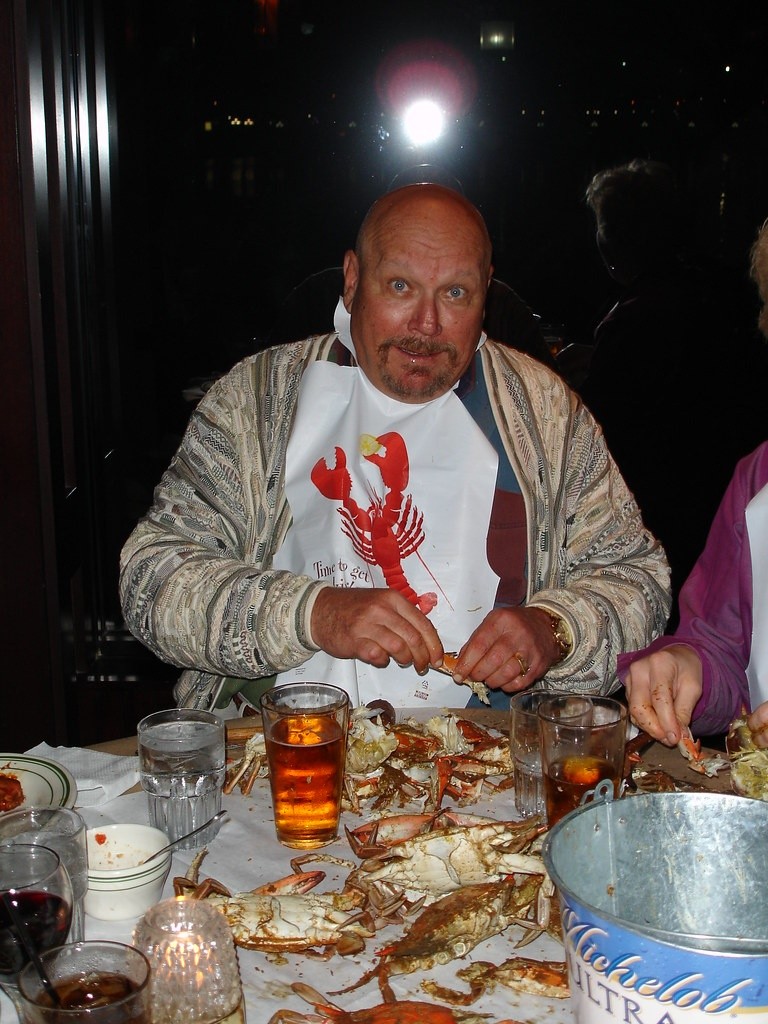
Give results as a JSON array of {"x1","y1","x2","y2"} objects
[{"x1": 531, "y1": 606, "x2": 574, "y2": 665}]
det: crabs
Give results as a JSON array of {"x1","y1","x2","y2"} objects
[{"x1": 173, "y1": 652, "x2": 768, "y2": 1024}]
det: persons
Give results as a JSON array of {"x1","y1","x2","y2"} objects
[
  {"x1": 271, "y1": 157, "x2": 768, "y2": 749},
  {"x1": 120, "y1": 184, "x2": 673, "y2": 715}
]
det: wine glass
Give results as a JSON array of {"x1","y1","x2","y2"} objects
[{"x1": 0, "y1": 844, "x2": 74, "y2": 1024}]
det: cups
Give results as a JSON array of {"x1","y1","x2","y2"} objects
[
  {"x1": 261, "y1": 683, "x2": 348, "y2": 852},
  {"x1": 18, "y1": 942, "x2": 150, "y2": 1024},
  {"x1": 509, "y1": 688, "x2": 594, "y2": 826},
  {"x1": 0, "y1": 806, "x2": 89, "y2": 944},
  {"x1": 138, "y1": 707, "x2": 227, "y2": 850},
  {"x1": 133, "y1": 898, "x2": 245, "y2": 1024},
  {"x1": 537, "y1": 694, "x2": 629, "y2": 832}
]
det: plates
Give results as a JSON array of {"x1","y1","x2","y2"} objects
[{"x1": 0, "y1": 753, "x2": 78, "y2": 810}]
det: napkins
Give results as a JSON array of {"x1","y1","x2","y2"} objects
[{"x1": 27, "y1": 742, "x2": 140, "y2": 810}]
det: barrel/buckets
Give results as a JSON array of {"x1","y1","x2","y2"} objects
[{"x1": 541, "y1": 780, "x2": 768, "y2": 1024}]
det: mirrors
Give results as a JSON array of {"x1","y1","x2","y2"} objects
[{"x1": 13, "y1": 0, "x2": 768, "y2": 718}]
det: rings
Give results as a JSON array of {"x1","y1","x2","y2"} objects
[{"x1": 511, "y1": 653, "x2": 531, "y2": 676}]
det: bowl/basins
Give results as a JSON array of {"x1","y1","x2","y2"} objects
[{"x1": 84, "y1": 824, "x2": 172, "y2": 922}]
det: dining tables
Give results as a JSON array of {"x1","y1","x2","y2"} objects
[{"x1": 0, "y1": 708, "x2": 768, "y2": 1024}]
